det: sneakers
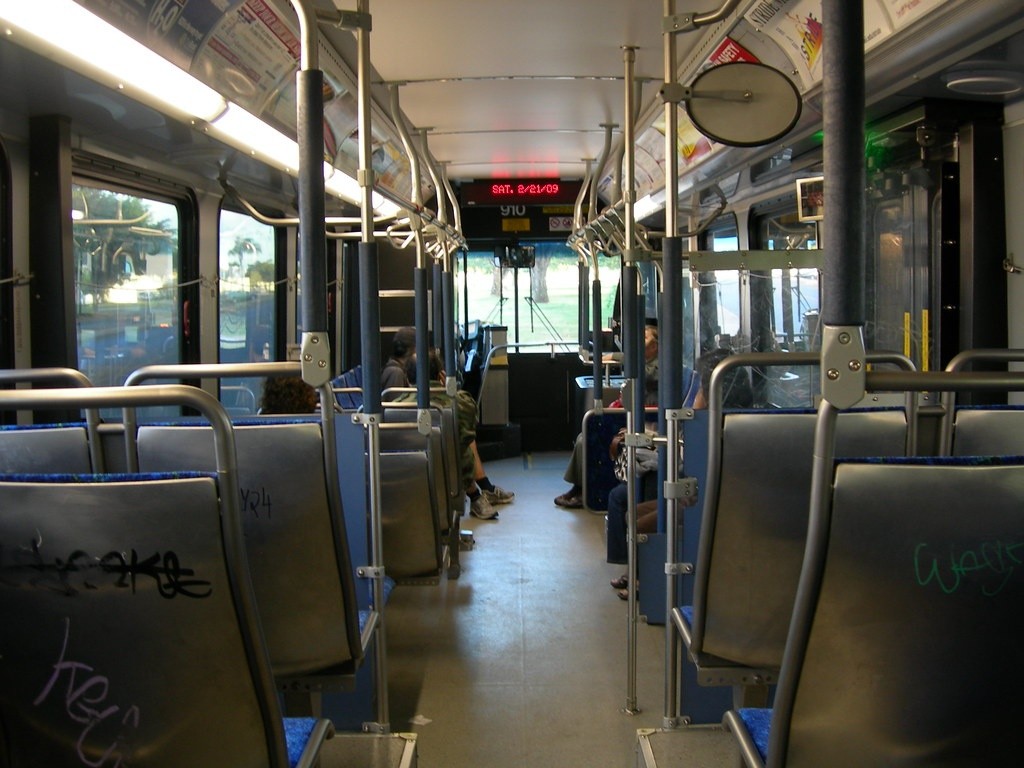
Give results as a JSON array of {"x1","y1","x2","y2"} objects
[
  {"x1": 481, "y1": 485, "x2": 514, "y2": 504},
  {"x1": 469, "y1": 495, "x2": 499, "y2": 519}
]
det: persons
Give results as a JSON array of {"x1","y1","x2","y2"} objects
[
  {"x1": 500, "y1": 252, "x2": 533, "y2": 269},
  {"x1": 604, "y1": 348, "x2": 753, "y2": 602},
  {"x1": 553, "y1": 316, "x2": 658, "y2": 509},
  {"x1": 379, "y1": 325, "x2": 513, "y2": 518},
  {"x1": 258, "y1": 376, "x2": 315, "y2": 415}
]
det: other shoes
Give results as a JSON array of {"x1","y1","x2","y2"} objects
[
  {"x1": 554, "y1": 494, "x2": 582, "y2": 508},
  {"x1": 610, "y1": 575, "x2": 627, "y2": 590},
  {"x1": 617, "y1": 588, "x2": 638, "y2": 600}
]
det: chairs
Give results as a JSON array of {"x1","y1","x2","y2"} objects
[
  {"x1": 631, "y1": 346, "x2": 1024, "y2": 766},
  {"x1": 1, "y1": 360, "x2": 455, "y2": 767}
]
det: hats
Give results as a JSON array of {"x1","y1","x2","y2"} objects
[{"x1": 395, "y1": 327, "x2": 416, "y2": 344}]
